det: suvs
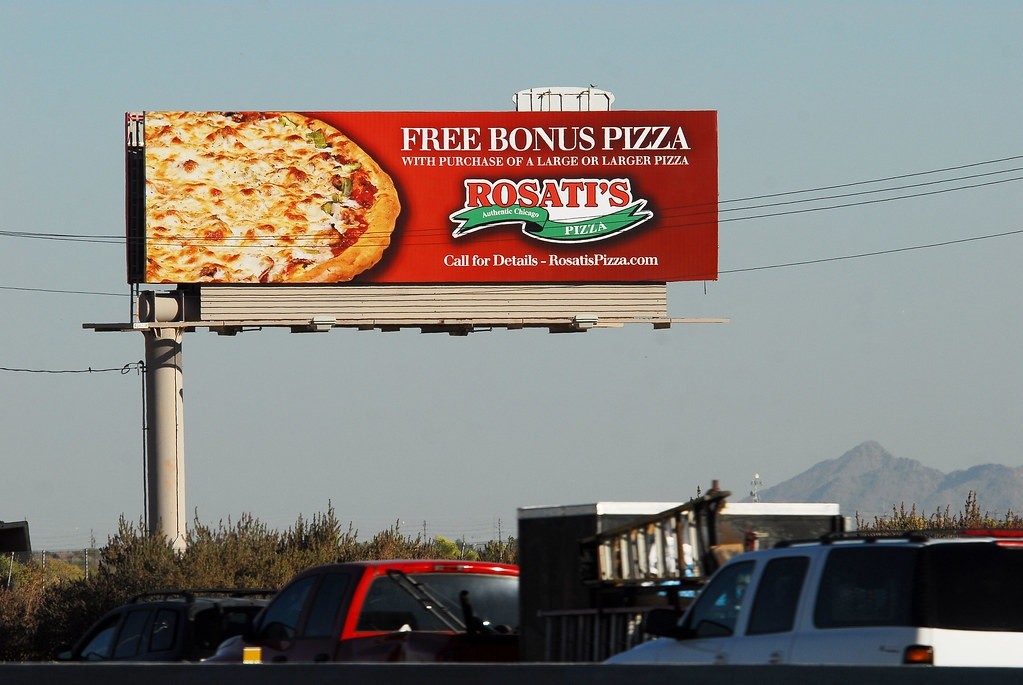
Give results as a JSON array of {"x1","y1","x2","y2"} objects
[
  {"x1": 201, "y1": 557, "x2": 525, "y2": 670},
  {"x1": 599, "y1": 527, "x2": 1023, "y2": 668},
  {"x1": 56, "y1": 586, "x2": 278, "y2": 663}
]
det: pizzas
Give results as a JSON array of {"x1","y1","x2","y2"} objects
[{"x1": 144, "y1": 111, "x2": 400, "y2": 283}]
